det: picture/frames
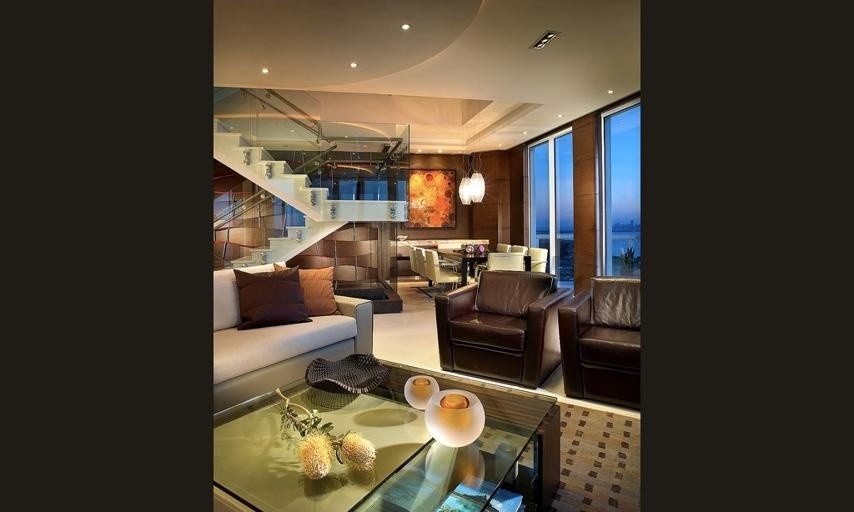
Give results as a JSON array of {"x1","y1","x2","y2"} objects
[{"x1": 401, "y1": 168, "x2": 457, "y2": 230}]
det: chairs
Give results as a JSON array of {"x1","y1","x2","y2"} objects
[
  {"x1": 434, "y1": 270, "x2": 574, "y2": 390},
  {"x1": 409, "y1": 243, "x2": 553, "y2": 293},
  {"x1": 558, "y1": 276, "x2": 641, "y2": 412}
]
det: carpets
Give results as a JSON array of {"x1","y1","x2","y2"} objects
[{"x1": 377, "y1": 359, "x2": 641, "y2": 512}]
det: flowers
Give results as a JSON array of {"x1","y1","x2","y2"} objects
[{"x1": 275, "y1": 388, "x2": 377, "y2": 480}]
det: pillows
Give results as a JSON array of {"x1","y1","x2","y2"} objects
[
  {"x1": 233, "y1": 265, "x2": 312, "y2": 331},
  {"x1": 273, "y1": 262, "x2": 343, "y2": 317}
]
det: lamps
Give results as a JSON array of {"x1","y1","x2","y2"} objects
[
  {"x1": 458, "y1": 155, "x2": 472, "y2": 205},
  {"x1": 471, "y1": 152, "x2": 486, "y2": 203}
]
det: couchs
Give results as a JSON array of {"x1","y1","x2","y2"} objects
[{"x1": 213, "y1": 262, "x2": 373, "y2": 415}]
